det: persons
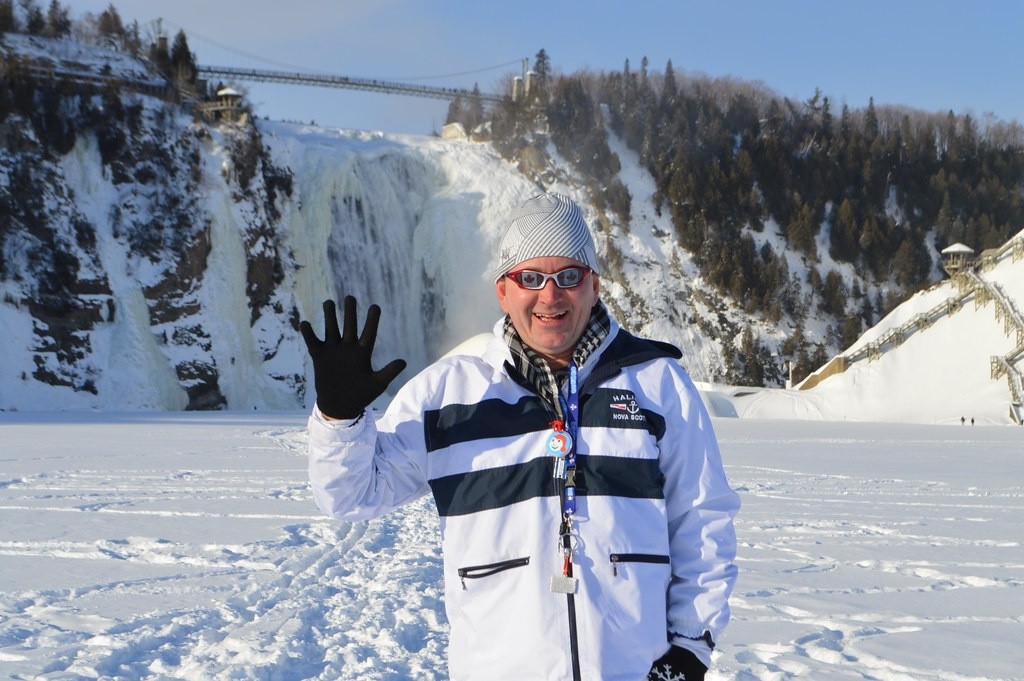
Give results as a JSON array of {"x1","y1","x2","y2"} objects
[{"x1": 301, "y1": 192, "x2": 742, "y2": 681}]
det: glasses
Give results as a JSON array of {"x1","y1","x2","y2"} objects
[{"x1": 505, "y1": 264, "x2": 592, "y2": 289}]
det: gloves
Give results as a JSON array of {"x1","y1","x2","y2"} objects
[
  {"x1": 647, "y1": 643, "x2": 711, "y2": 680},
  {"x1": 298, "y1": 295, "x2": 405, "y2": 420}
]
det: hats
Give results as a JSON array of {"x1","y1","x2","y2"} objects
[{"x1": 492, "y1": 191, "x2": 601, "y2": 284}]
